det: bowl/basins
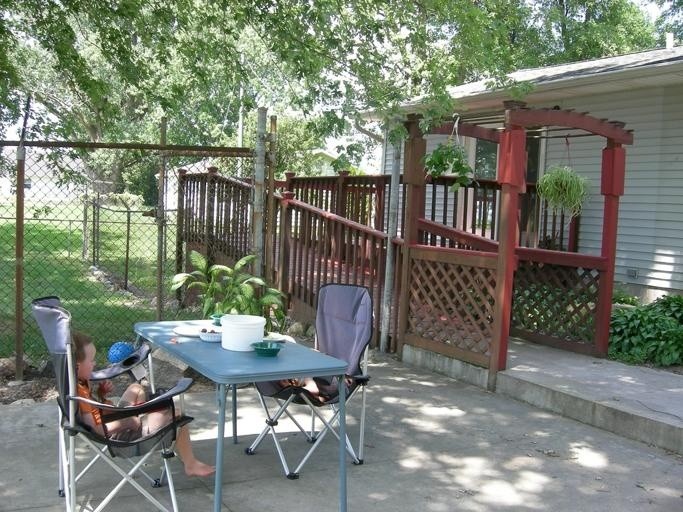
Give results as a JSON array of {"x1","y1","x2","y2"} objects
[
  {"x1": 251, "y1": 340, "x2": 284, "y2": 357},
  {"x1": 209, "y1": 314, "x2": 225, "y2": 326},
  {"x1": 200, "y1": 331, "x2": 222, "y2": 343}
]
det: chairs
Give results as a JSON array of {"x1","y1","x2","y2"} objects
[
  {"x1": 246, "y1": 282, "x2": 375, "y2": 481},
  {"x1": 28, "y1": 293, "x2": 193, "y2": 512}
]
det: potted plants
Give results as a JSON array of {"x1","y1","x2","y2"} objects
[{"x1": 423, "y1": 139, "x2": 478, "y2": 192}]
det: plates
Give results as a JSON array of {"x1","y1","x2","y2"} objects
[{"x1": 172, "y1": 326, "x2": 202, "y2": 337}]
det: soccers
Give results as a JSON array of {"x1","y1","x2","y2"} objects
[{"x1": 108, "y1": 342, "x2": 136, "y2": 363}]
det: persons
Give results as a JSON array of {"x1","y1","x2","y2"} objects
[{"x1": 69, "y1": 330, "x2": 217, "y2": 478}]
